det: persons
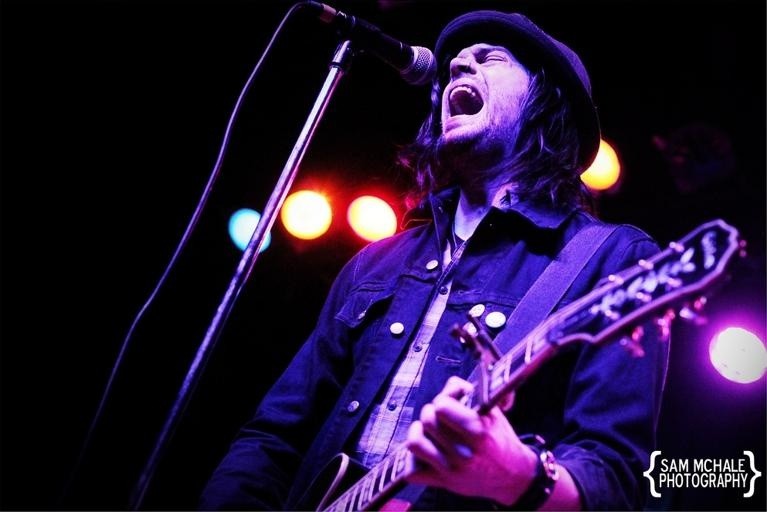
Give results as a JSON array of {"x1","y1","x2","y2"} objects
[{"x1": 196, "y1": 10, "x2": 672, "y2": 511}]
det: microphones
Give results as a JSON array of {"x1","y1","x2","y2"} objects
[{"x1": 306, "y1": 0, "x2": 438, "y2": 87}]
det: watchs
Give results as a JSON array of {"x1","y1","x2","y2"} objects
[{"x1": 509, "y1": 434, "x2": 558, "y2": 511}]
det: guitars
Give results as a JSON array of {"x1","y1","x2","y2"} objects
[{"x1": 295, "y1": 219, "x2": 747, "y2": 512}]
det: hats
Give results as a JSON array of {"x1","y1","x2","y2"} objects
[{"x1": 433, "y1": 10, "x2": 601, "y2": 176}]
